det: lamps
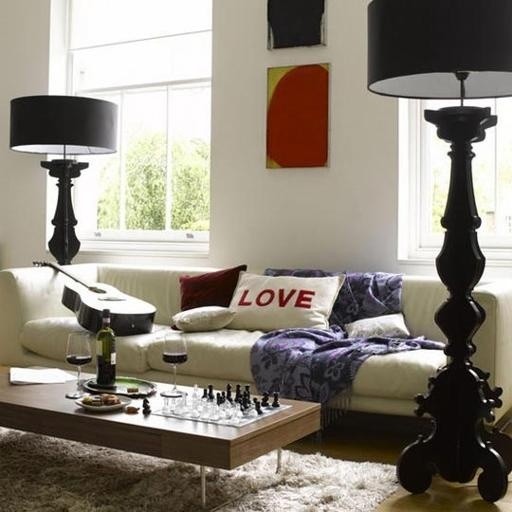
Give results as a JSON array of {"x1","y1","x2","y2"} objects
[
  {"x1": 8, "y1": 89, "x2": 118, "y2": 263},
  {"x1": 365, "y1": 2, "x2": 512, "y2": 503}
]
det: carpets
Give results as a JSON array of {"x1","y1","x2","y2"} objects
[{"x1": 1, "y1": 427, "x2": 401, "y2": 511}]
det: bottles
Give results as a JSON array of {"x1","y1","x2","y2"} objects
[{"x1": 95, "y1": 308, "x2": 118, "y2": 385}]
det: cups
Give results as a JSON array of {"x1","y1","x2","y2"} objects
[
  {"x1": 160, "y1": 331, "x2": 190, "y2": 398},
  {"x1": 67, "y1": 331, "x2": 93, "y2": 399}
]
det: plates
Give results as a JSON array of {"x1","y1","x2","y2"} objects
[{"x1": 72, "y1": 395, "x2": 131, "y2": 411}]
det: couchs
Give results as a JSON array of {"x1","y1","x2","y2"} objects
[{"x1": 1, "y1": 263, "x2": 511, "y2": 445}]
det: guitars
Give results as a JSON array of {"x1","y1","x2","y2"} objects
[{"x1": 32, "y1": 261, "x2": 157, "y2": 335}]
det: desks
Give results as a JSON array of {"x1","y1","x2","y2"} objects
[{"x1": 1, "y1": 364, "x2": 323, "y2": 511}]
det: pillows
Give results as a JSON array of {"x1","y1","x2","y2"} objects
[
  {"x1": 344, "y1": 313, "x2": 411, "y2": 342},
  {"x1": 224, "y1": 269, "x2": 345, "y2": 334},
  {"x1": 179, "y1": 262, "x2": 247, "y2": 313},
  {"x1": 170, "y1": 306, "x2": 236, "y2": 331}
]
